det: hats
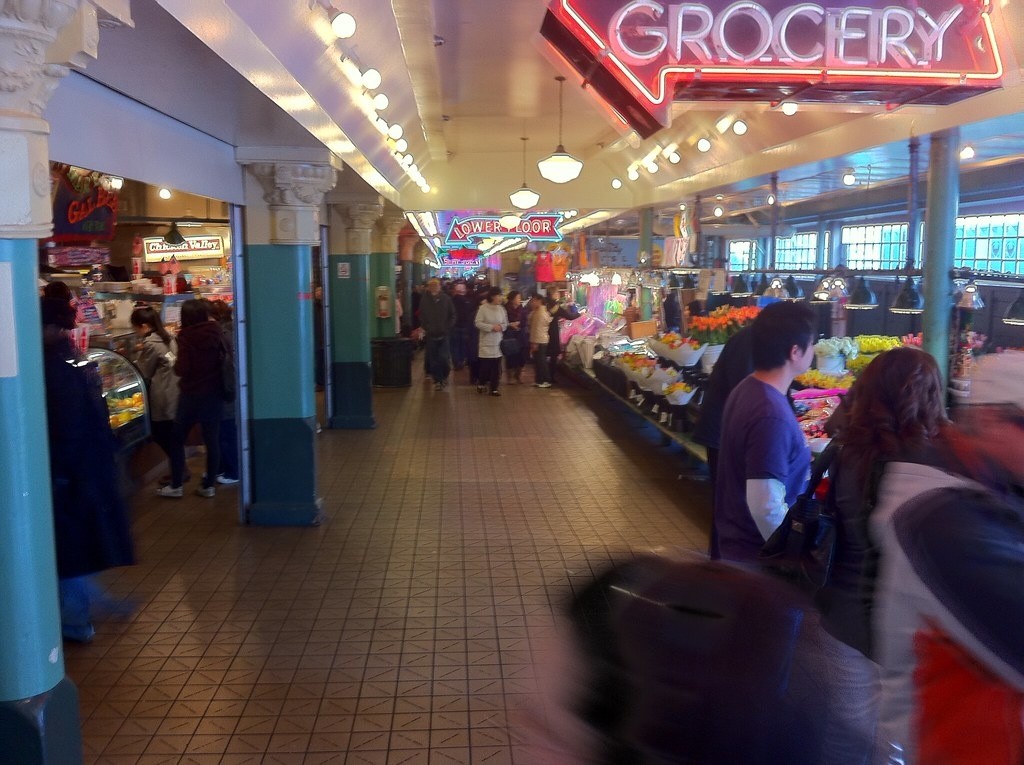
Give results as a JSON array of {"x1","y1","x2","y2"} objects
[
  {"x1": 35, "y1": 280, "x2": 78, "y2": 331},
  {"x1": 954, "y1": 349, "x2": 1024, "y2": 409},
  {"x1": 427, "y1": 278, "x2": 441, "y2": 285}
]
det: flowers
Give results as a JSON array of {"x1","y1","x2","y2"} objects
[
  {"x1": 792, "y1": 332, "x2": 924, "y2": 478},
  {"x1": 615, "y1": 303, "x2": 762, "y2": 434}
]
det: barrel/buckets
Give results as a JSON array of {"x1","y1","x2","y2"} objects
[{"x1": 631, "y1": 321, "x2": 657, "y2": 340}]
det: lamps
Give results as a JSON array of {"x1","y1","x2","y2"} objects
[
  {"x1": 309, "y1": 0, "x2": 356, "y2": 39},
  {"x1": 163, "y1": 220, "x2": 185, "y2": 247},
  {"x1": 341, "y1": 44, "x2": 382, "y2": 89},
  {"x1": 843, "y1": 164, "x2": 879, "y2": 310},
  {"x1": 888, "y1": 144, "x2": 925, "y2": 314},
  {"x1": 509, "y1": 137, "x2": 540, "y2": 209},
  {"x1": 360, "y1": 87, "x2": 390, "y2": 109},
  {"x1": 957, "y1": 279, "x2": 986, "y2": 309},
  {"x1": 1002, "y1": 288, "x2": 1024, "y2": 326},
  {"x1": 536, "y1": 76, "x2": 583, "y2": 183},
  {"x1": 626, "y1": 270, "x2": 847, "y2": 302}
]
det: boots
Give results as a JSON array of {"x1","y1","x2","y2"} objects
[
  {"x1": 515, "y1": 367, "x2": 524, "y2": 385},
  {"x1": 506, "y1": 368, "x2": 516, "y2": 384}
]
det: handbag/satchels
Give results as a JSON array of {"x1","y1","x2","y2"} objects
[
  {"x1": 500, "y1": 323, "x2": 519, "y2": 357},
  {"x1": 758, "y1": 432, "x2": 849, "y2": 590}
]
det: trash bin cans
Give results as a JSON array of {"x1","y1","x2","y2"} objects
[{"x1": 370, "y1": 337, "x2": 415, "y2": 388}]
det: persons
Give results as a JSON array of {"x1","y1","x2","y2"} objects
[
  {"x1": 130, "y1": 304, "x2": 191, "y2": 481},
  {"x1": 526, "y1": 547, "x2": 882, "y2": 764},
  {"x1": 767, "y1": 354, "x2": 966, "y2": 656},
  {"x1": 869, "y1": 350, "x2": 1024, "y2": 765},
  {"x1": 40, "y1": 280, "x2": 139, "y2": 640},
  {"x1": 701, "y1": 300, "x2": 818, "y2": 561},
  {"x1": 313, "y1": 286, "x2": 325, "y2": 392},
  {"x1": 395, "y1": 276, "x2": 584, "y2": 396},
  {"x1": 157, "y1": 298, "x2": 238, "y2": 497}
]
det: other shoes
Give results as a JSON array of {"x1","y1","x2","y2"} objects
[
  {"x1": 488, "y1": 390, "x2": 501, "y2": 396},
  {"x1": 217, "y1": 473, "x2": 241, "y2": 484},
  {"x1": 195, "y1": 472, "x2": 216, "y2": 498},
  {"x1": 536, "y1": 381, "x2": 552, "y2": 388},
  {"x1": 476, "y1": 386, "x2": 487, "y2": 393},
  {"x1": 314, "y1": 383, "x2": 324, "y2": 392},
  {"x1": 159, "y1": 467, "x2": 192, "y2": 483},
  {"x1": 153, "y1": 485, "x2": 183, "y2": 498},
  {"x1": 434, "y1": 382, "x2": 442, "y2": 390},
  {"x1": 59, "y1": 623, "x2": 95, "y2": 644}
]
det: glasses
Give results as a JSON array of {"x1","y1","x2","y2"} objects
[{"x1": 994, "y1": 413, "x2": 1024, "y2": 430}]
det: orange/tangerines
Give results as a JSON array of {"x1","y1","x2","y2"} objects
[{"x1": 108, "y1": 392, "x2": 142, "y2": 430}]
